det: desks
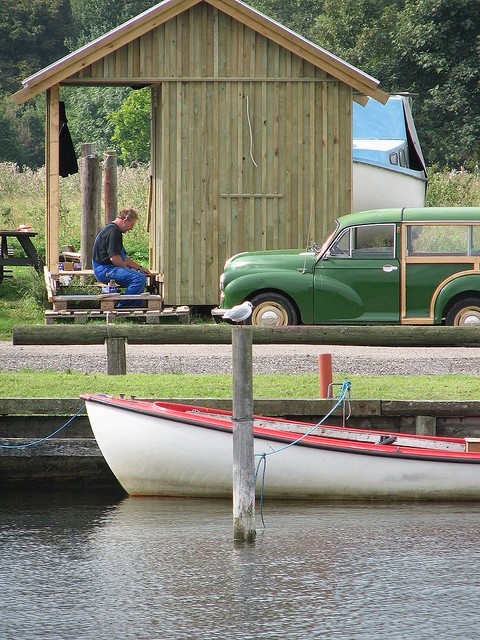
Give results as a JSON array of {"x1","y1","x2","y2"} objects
[{"x1": 0, "y1": 229, "x2": 46, "y2": 284}]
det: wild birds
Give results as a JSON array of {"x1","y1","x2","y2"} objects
[{"x1": 211, "y1": 301, "x2": 255, "y2": 328}]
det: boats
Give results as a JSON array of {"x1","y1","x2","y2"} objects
[{"x1": 80, "y1": 395, "x2": 480, "y2": 500}]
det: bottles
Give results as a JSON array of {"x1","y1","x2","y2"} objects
[{"x1": 110, "y1": 280, "x2": 116, "y2": 292}]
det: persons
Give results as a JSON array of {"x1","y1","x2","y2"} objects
[{"x1": 92, "y1": 208, "x2": 151, "y2": 308}]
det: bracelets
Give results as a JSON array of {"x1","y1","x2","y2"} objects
[{"x1": 137, "y1": 265, "x2": 142, "y2": 272}]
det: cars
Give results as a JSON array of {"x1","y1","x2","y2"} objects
[{"x1": 211, "y1": 207, "x2": 480, "y2": 326}]
